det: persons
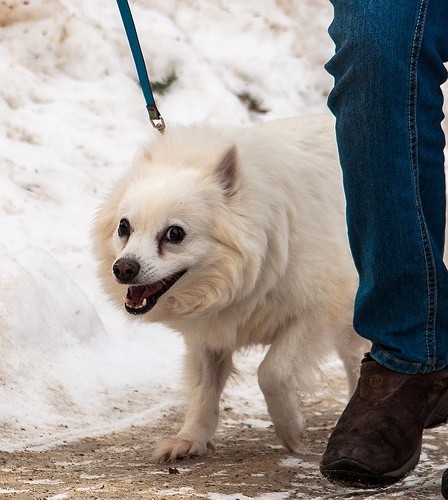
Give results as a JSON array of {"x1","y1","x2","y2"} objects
[{"x1": 304, "y1": 2, "x2": 447, "y2": 499}]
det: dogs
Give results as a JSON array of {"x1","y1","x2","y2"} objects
[{"x1": 87, "y1": 111, "x2": 372, "y2": 464}]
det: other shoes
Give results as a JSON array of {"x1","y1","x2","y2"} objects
[{"x1": 319, "y1": 352, "x2": 448, "y2": 487}]
